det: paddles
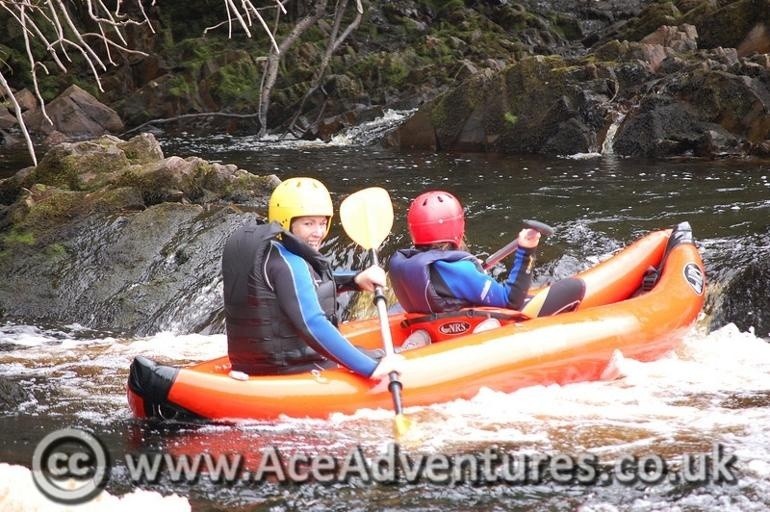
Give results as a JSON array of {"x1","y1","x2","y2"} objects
[
  {"x1": 352, "y1": 220, "x2": 554, "y2": 319},
  {"x1": 339, "y1": 187, "x2": 412, "y2": 439}
]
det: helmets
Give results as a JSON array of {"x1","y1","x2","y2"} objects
[
  {"x1": 269, "y1": 176, "x2": 333, "y2": 244},
  {"x1": 409, "y1": 190, "x2": 464, "y2": 248}
]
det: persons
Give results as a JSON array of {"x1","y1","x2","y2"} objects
[
  {"x1": 223, "y1": 177, "x2": 502, "y2": 379},
  {"x1": 390, "y1": 191, "x2": 586, "y2": 343}
]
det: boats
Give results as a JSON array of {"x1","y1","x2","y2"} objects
[{"x1": 127, "y1": 221, "x2": 705, "y2": 426}]
